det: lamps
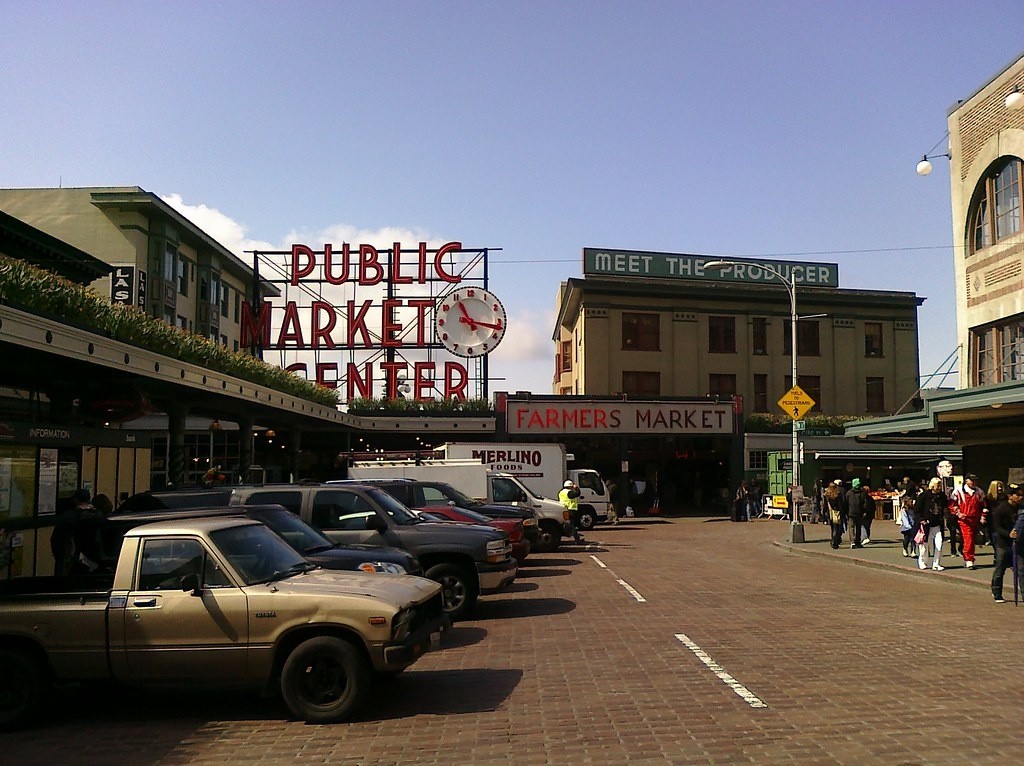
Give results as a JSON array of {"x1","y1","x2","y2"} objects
[
  {"x1": 916, "y1": 148, "x2": 952, "y2": 175},
  {"x1": 1006, "y1": 85, "x2": 1024, "y2": 110}
]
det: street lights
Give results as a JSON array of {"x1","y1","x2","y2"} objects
[{"x1": 703, "y1": 260, "x2": 805, "y2": 544}]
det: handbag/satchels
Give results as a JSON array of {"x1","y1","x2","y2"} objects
[
  {"x1": 829, "y1": 509, "x2": 841, "y2": 525},
  {"x1": 914, "y1": 523, "x2": 925, "y2": 544}
]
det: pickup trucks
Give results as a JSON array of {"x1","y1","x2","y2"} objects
[
  {"x1": 0, "y1": 516, "x2": 454, "y2": 734},
  {"x1": 113, "y1": 486, "x2": 519, "y2": 622}
]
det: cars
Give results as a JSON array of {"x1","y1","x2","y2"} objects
[{"x1": 337, "y1": 503, "x2": 531, "y2": 562}]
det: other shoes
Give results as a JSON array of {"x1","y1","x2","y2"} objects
[
  {"x1": 850, "y1": 543, "x2": 859, "y2": 548},
  {"x1": 965, "y1": 562, "x2": 975, "y2": 570},
  {"x1": 932, "y1": 564, "x2": 945, "y2": 571},
  {"x1": 902, "y1": 550, "x2": 909, "y2": 557},
  {"x1": 911, "y1": 552, "x2": 918, "y2": 557},
  {"x1": 957, "y1": 549, "x2": 963, "y2": 558},
  {"x1": 992, "y1": 593, "x2": 1005, "y2": 603},
  {"x1": 830, "y1": 543, "x2": 840, "y2": 549},
  {"x1": 951, "y1": 555, "x2": 956, "y2": 557},
  {"x1": 918, "y1": 563, "x2": 929, "y2": 570},
  {"x1": 862, "y1": 538, "x2": 870, "y2": 544}
]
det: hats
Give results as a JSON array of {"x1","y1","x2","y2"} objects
[
  {"x1": 1008, "y1": 483, "x2": 1021, "y2": 493},
  {"x1": 964, "y1": 472, "x2": 976, "y2": 479},
  {"x1": 834, "y1": 479, "x2": 843, "y2": 486},
  {"x1": 852, "y1": 478, "x2": 861, "y2": 487}
]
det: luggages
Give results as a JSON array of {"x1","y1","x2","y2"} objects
[{"x1": 731, "y1": 499, "x2": 742, "y2": 522}]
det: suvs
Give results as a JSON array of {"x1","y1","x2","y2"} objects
[
  {"x1": 326, "y1": 479, "x2": 543, "y2": 551},
  {"x1": 107, "y1": 502, "x2": 424, "y2": 582}
]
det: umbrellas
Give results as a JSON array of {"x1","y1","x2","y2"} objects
[{"x1": 1013, "y1": 531, "x2": 1018, "y2": 607}]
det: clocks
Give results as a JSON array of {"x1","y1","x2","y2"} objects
[{"x1": 433, "y1": 285, "x2": 507, "y2": 358}]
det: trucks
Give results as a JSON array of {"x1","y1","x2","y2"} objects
[
  {"x1": 431, "y1": 441, "x2": 611, "y2": 531},
  {"x1": 347, "y1": 460, "x2": 572, "y2": 555}
]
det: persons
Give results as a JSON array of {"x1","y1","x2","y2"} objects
[
  {"x1": 558, "y1": 480, "x2": 582, "y2": 544},
  {"x1": 824, "y1": 478, "x2": 874, "y2": 549},
  {"x1": 989, "y1": 483, "x2": 1023, "y2": 606},
  {"x1": 810, "y1": 479, "x2": 829, "y2": 525},
  {"x1": 899, "y1": 477, "x2": 1007, "y2": 571},
  {"x1": 605, "y1": 480, "x2": 620, "y2": 526},
  {"x1": 50, "y1": 488, "x2": 113, "y2": 575},
  {"x1": 736, "y1": 478, "x2": 762, "y2": 522}
]
